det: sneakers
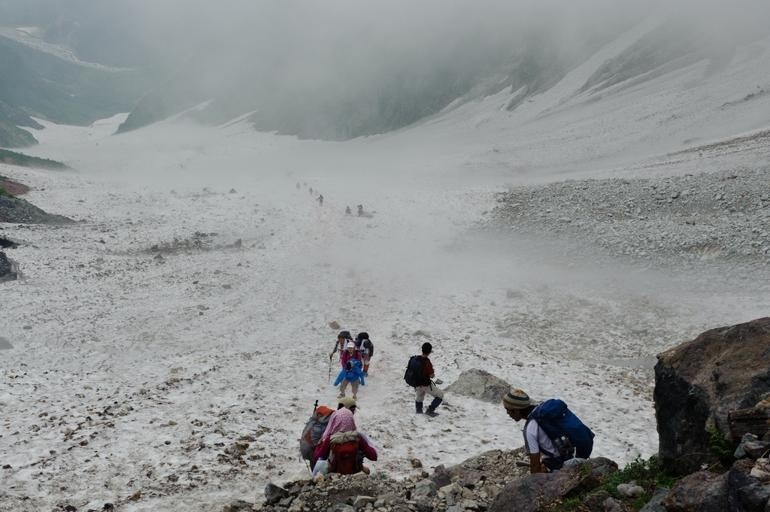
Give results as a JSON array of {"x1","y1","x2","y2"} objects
[
  {"x1": 337, "y1": 393, "x2": 345, "y2": 399},
  {"x1": 363, "y1": 372, "x2": 368, "y2": 376},
  {"x1": 353, "y1": 395, "x2": 357, "y2": 400}
]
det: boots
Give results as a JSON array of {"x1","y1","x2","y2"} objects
[
  {"x1": 425, "y1": 397, "x2": 442, "y2": 417},
  {"x1": 416, "y1": 401, "x2": 423, "y2": 413}
]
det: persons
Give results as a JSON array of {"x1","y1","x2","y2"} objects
[
  {"x1": 312, "y1": 408, "x2": 377, "y2": 475},
  {"x1": 333, "y1": 357, "x2": 365, "y2": 400},
  {"x1": 329, "y1": 331, "x2": 353, "y2": 359},
  {"x1": 502, "y1": 388, "x2": 573, "y2": 474},
  {"x1": 310, "y1": 396, "x2": 378, "y2": 476},
  {"x1": 414, "y1": 342, "x2": 443, "y2": 417},
  {"x1": 341, "y1": 342, "x2": 362, "y2": 367},
  {"x1": 354, "y1": 332, "x2": 373, "y2": 377}
]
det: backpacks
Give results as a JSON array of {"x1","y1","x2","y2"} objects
[
  {"x1": 404, "y1": 355, "x2": 436, "y2": 391},
  {"x1": 300, "y1": 406, "x2": 334, "y2": 460},
  {"x1": 355, "y1": 332, "x2": 369, "y2": 346},
  {"x1": 340, "y1": 332, "x2": 352, "y2": 340},
  {"x1": 526, "y1": 398, "x2": 594, "y2": 456}
]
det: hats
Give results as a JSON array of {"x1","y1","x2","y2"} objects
[
  {"x1": 347, "y1": 342, "x2": 355, "y2": 347},
  {"x1": 422, "y1": 342, "x2": 433, "y2": 352},
  {"x1": 503, "y1": 389, "x2": 530, "y2": 409}
]
